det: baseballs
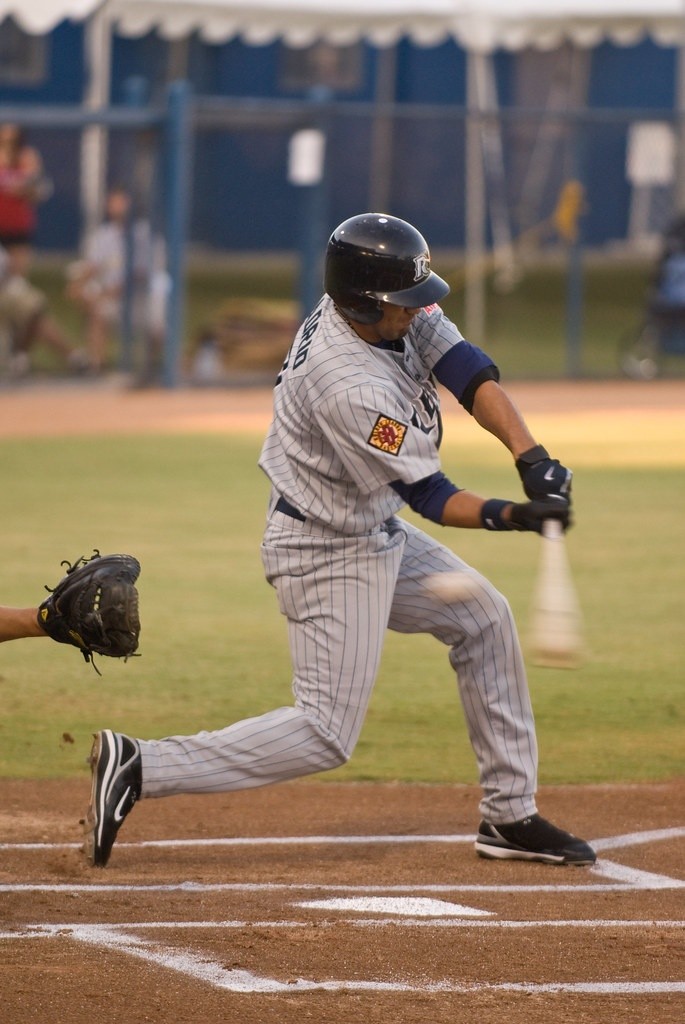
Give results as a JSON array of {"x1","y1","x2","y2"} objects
[{"x1": 431, "y1": 569, "x2": 479, "y2": 602}]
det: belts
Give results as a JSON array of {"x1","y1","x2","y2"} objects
[{"x1": 274, "y1": 497, "x2": 307, "y2": 523}]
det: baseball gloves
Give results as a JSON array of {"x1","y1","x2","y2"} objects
[{"x1": 39, "y1": 554, "x2": 142, "y2": 659}]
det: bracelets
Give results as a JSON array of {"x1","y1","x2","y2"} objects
[{"x1": 481, "y1": 498, "x2": 514, "y2": 531}]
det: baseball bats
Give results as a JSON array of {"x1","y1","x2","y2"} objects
[{"x1": 528, "y1": 519, "x2": 588, "y2": 669}]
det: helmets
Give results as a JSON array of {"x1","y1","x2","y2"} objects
[{"x1": 323, "y1": 212, "x2": 451, "y2": 325}]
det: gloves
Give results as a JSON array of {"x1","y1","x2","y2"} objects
[
  {"x1": 480, "y1": 498, "x2": 569, "y2": 537},
  {"x1": 514, "y1": 444, "x2": 573, "y2": 505}
]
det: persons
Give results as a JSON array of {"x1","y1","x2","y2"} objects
[
  {"x1": 64, "y1": 189, "x2": 167, "y2": 373},
  {"x1": 0, "y1": 553, "x2": 142, "y2": 658},
  {"x1": 79, "y1": 212, "x2": 598, "y2": 870},
  {"x1": 0, "y1": 121, "x2": 53, "y2": 245}
]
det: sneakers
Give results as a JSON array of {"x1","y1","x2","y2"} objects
[
  {"x1": 474, "y1": 813, "x2": 598, "y2": 865},
  {"x1": 80, "y1": 729, "x2": 142, "y2": 869}
]
